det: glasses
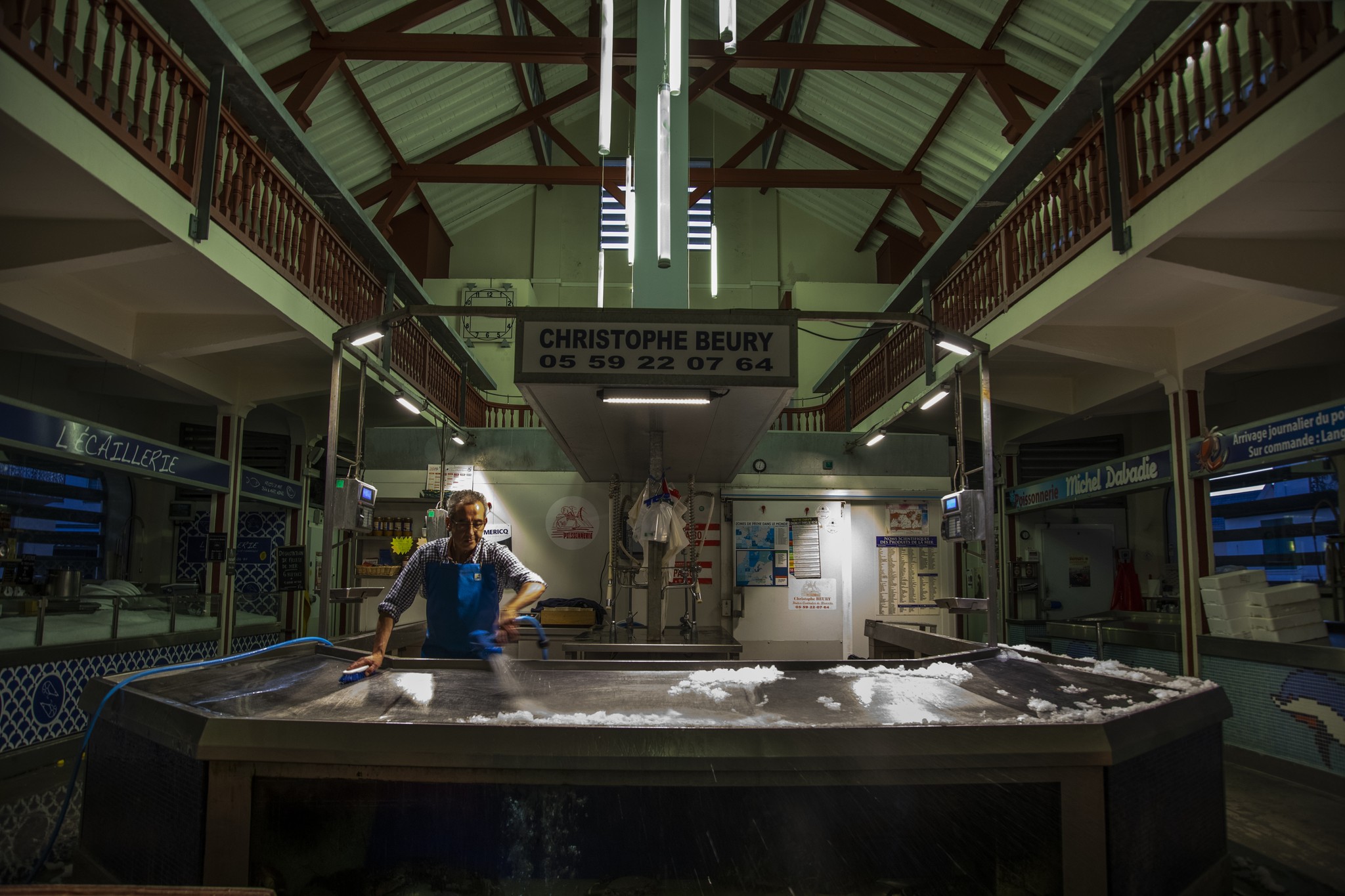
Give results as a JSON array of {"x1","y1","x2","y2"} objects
[{"x1": 450, "y1": 518, "x2": 485, "y2": 531}]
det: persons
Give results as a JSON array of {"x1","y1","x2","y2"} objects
[{"x1": 346, "y1": 489, "x2": 547, "y2": 677}]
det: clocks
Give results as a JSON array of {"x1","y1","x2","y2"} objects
[{"x1": 460, "y1": 282, "x2": 519, "y2": 349}]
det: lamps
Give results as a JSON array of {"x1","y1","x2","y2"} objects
[
  {"x1": 932, "y1": 332, "x2": 976, "y2": 356},
  {"x1": 451, "y1": 431, "x2": 467, "y2": 445},
  {"x1": 595, "y1": 388, "x2": 729, "y2": 404},
  {"x1": 917, "y1": 383, "x2": 951, "y2": 410},
  {"x1": 394, "y1": 390, "x2": 423, "y2": 414},
  {"x1": 348, "y1": 322, "x2": 389, "y2": 346},
  {"x1": 863, "y1": 428, "x2": 887, "y2": 446}
]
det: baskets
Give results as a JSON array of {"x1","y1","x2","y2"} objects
[{"x1": 354, "y1": 565, "x2": 401, "y2": 577}]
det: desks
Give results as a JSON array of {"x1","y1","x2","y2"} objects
[{"x1": 562, "y1": 624, "x2": 744, "y2": 659}]
